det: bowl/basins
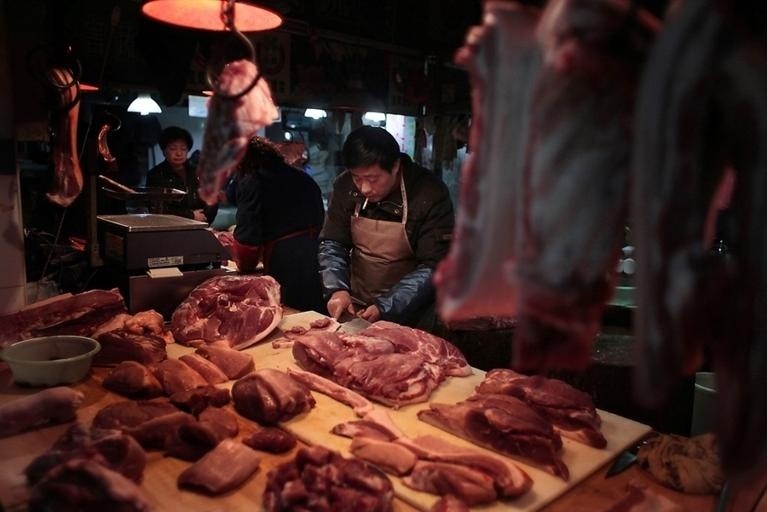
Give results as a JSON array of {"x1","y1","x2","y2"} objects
[
  {"x1": 0, "y1": 333, "x2": 102, "y2": 388},
  {"x1": 608, "y1": 285, "x2": 637, "y2": 306}
]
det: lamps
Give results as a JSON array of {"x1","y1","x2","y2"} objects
[
  {"x1": 126, "y1": 93, "x2": 162, "y2": 117},
  {"x1": 141, "y1": 0, "x2": 284, "y2": 33}
]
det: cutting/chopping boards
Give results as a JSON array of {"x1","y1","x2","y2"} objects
[{"x1": 166, "y1": 309, "x2": 656, "y2": 512}]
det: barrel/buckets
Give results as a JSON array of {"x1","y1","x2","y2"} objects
[{"x1": 691, "y1": 371, "x2": 718, "y2": 437}]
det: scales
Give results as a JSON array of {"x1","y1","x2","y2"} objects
[{"x1": 96, "y1": 213, "x2": 233, "y2": 271}]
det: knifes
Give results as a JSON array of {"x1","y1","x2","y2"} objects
[
  {"x1": 606, "y1": 437, "x2": 648, "y2": 479},
  {"x1": 335, "y1": 312, "x2": 373, "y2": 337}
]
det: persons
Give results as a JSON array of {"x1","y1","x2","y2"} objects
[
  {"x1": 147, "y1": 127, "x2": 217, "y2": 226},
  {"x1": 317, "y1": 126, "x2": 452, "y2": 345},
  {"x1": 229, "y1": 137, "x2": 322, "y2": 311}
]
